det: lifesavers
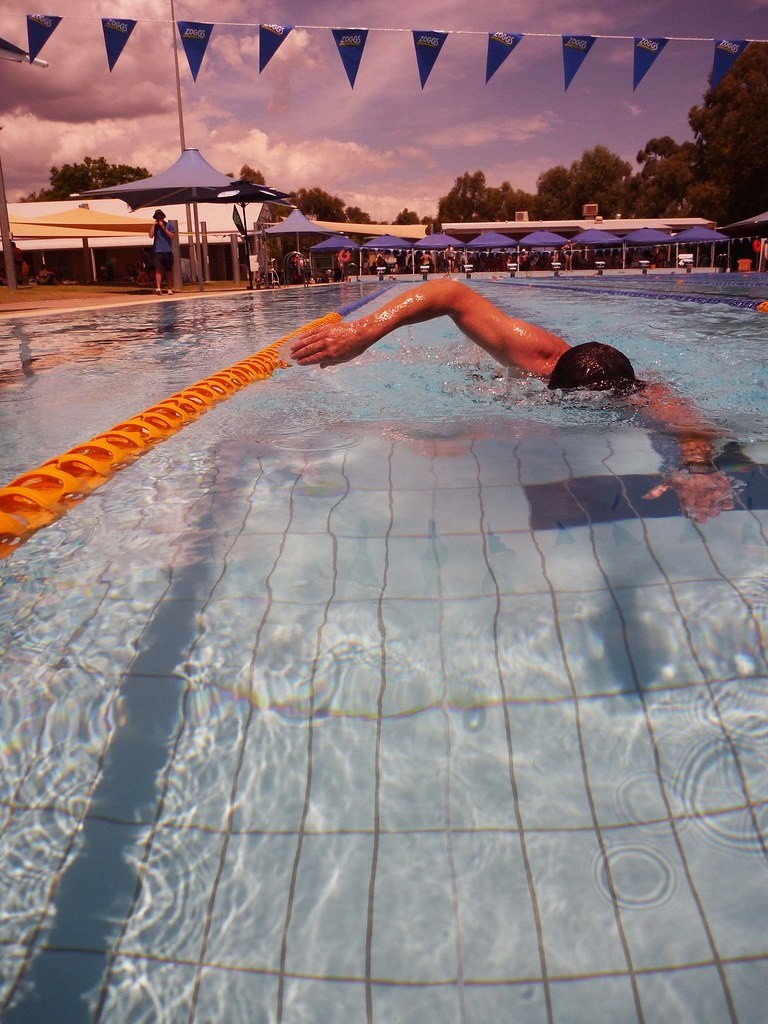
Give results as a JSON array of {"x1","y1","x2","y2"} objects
[
  {"x1": 752, "y1": 239, "x2": 761, "y2": 253},
  {"x1": 338, "y1": 250, "x2": 351, "y2": 262}
]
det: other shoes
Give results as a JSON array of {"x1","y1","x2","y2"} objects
[
  {"x1": 156, "y1": 288, "x2": 162, "y2": 295},
  {"x1": 168, "y1": 289, "x2": 174, "y2": 295}
]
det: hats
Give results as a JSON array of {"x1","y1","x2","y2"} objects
[{"x1": 153, "y1": 209, "x2": 166, "y2": 220}]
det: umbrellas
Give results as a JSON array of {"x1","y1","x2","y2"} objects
[
  {"x1": 716, "y1": 211, "x2": 768, "y2": 231},
  {"x1": 71, "y1": 147, "x2": 294, "y2": 287},
  {"x1": 242, "y1": 208, "x2": 349, "y2": 274}
]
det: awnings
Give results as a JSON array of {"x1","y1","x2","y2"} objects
[
  {"x1": 262, "y1": 216, "x2": 426, "y2": 239},
  {"x1": 0, "y1": 209, "x2": 242, "y2": 240}
]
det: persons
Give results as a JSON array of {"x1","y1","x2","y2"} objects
[
  {"x1": 12, "y1": 242, "x2": 29, "y2": 285},
  {"x1": 135, "y1": 264, "x2": 164, "y2": 286},
  {"x1": 290, "y1": 279, "x2": 636, "y2": 391},
  {"x1": 36, "y1": 265, "x2": 62, "y2": 286},
  {"x1": 150, "y1": 209, "x2": 175, "y2": 294},
  {"x1": 256, "y1": 235, "x2": 768, "y2": 289}
]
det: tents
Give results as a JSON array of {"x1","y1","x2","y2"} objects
[{"x1": 309, "y1": 226, "x2": 731, "y2": 269}]
